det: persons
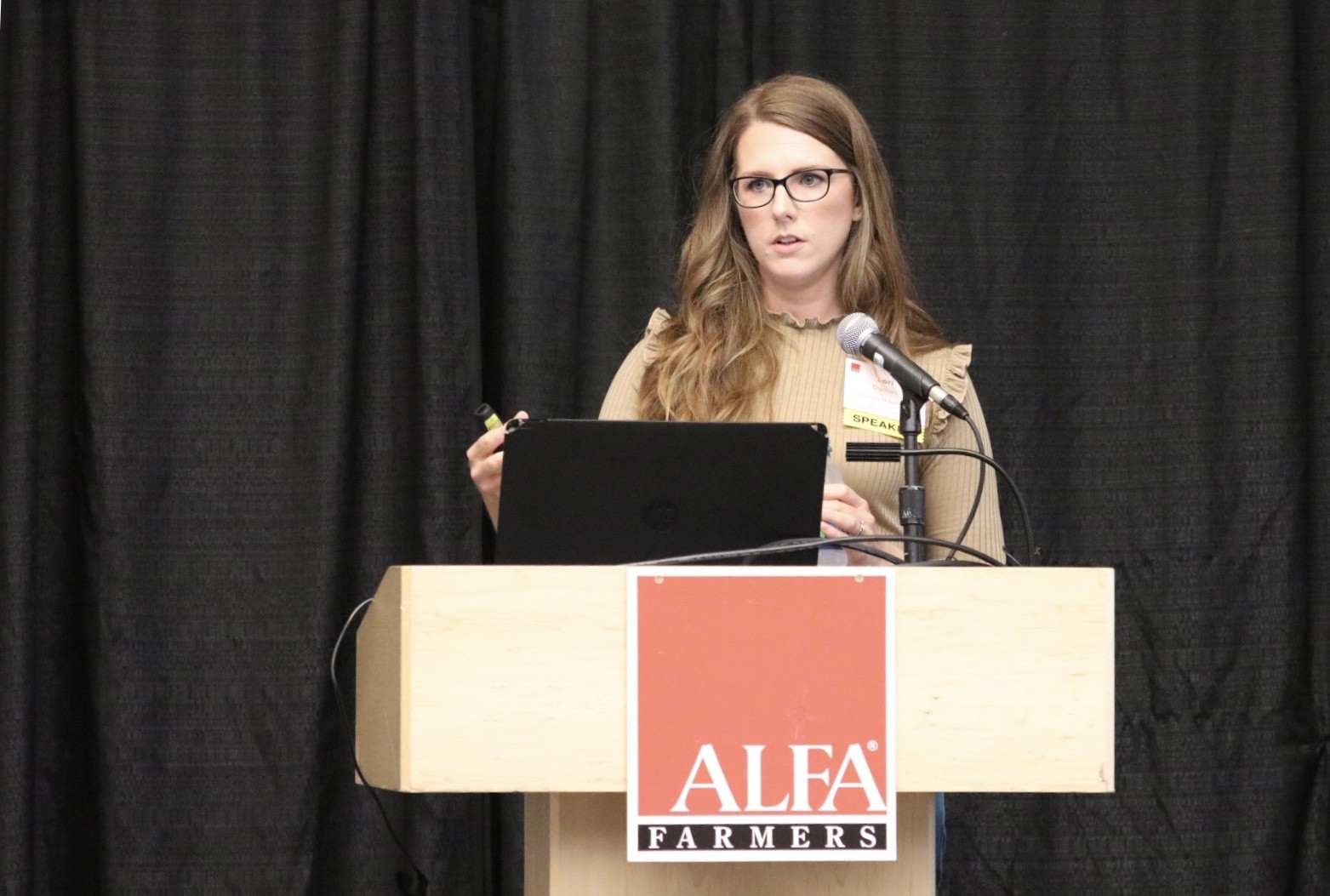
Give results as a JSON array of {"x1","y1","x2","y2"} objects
[{"x1": 467, "y1": 71, "x2": 1005, "y2": 884}]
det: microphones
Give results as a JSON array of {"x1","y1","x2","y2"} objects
[
  {"x1": 835, "y1": 310, "x2": 968, "y2": 420},
  {"x1": 844, "y1": 442, "x2": 1037, "y2": 566}
]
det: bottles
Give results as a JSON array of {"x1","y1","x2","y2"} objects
[{"x1": 819, "y1": 448, "x2": 850, "y2": 567}]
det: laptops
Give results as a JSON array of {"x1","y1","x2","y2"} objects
[{"x1": 494, "y1": 418, "x2": 828, "y2": 567}]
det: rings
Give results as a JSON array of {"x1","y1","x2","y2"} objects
[{"x1": 849, "y1": 519, "x2": 864, "y2": 537}]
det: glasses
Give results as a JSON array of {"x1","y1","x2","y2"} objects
[{"x1": 730, "y1": 167, "x2": 854, "y2": 209}]
